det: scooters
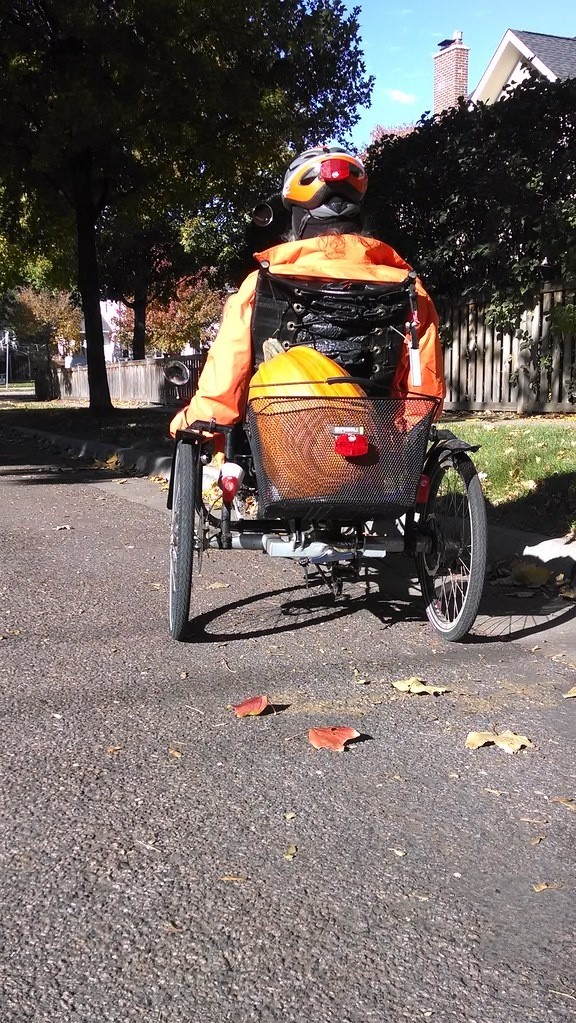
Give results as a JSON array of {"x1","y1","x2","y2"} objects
[{"x1": 161, "y1": 260, "x2": 488, "y2": 642}]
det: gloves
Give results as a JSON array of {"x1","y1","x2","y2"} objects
[{"x1": 170, "y1": 405, "x2": 210, "y2": 444}]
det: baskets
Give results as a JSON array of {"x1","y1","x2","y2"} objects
[{"x1": 240, "y1": 394, "x2": 444, "y2": 522}]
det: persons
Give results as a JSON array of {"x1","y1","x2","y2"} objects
[{"x1": 170, "y1": 146, "x2": 446, "y2": 468}]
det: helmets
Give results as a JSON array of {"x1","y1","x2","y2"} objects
[{"x1": 281, "y1": 146, "x2": 368, "y2": 210}]
